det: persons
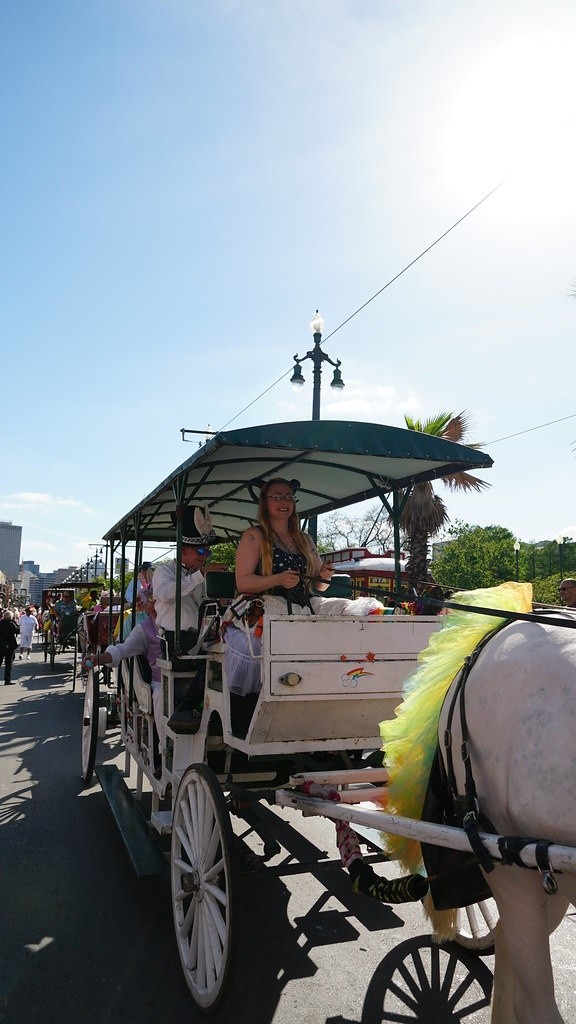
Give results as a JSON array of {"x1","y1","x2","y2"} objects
[
  {"x1": 223, "y1": 478, "x2": 384, "y2": 695},
  {"x1": 0, "y1": 594, "x2": 76, "y2": 659},
  {"x1": 124, "y1": 562, "x2": 152, "y2": 604},
  {"x1": 81, "y1": 589, "x2": 163, "y2": 756},
  {"x1": 152, "y1": 504, "x2": 227, "y2": 671},
  {"x1": 0, "y1": 611, "x2": 20, "y2": 685},
  {"x1": 81, "y1": 589, "x2": 120, "y2": 613},
  {"x1": 559, "y1": 578, "x2": 576, "y2": 609}
]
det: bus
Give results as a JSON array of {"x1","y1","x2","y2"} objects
[{"x1": 319, "y1": 547, "x2": 432, "y2": 607}]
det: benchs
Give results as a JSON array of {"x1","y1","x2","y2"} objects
[{"x1": 74, "y1": 568, "x2": 441, "y2": 778}]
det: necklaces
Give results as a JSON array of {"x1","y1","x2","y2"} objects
[{"x1": 273, "y1": 528, "x2": 306, "y2": 595}]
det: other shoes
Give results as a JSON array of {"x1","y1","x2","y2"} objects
[
  {"x1": 26, "y1": 656, "x2": 30, "y2": 659},
  {"x1": 167, "y1": 709, "x2": 201, "y2": 734},
  {"x1": 5, "y1": 681, "x2": 16, "y2": 685},
  {"x1": 19, "y1": 655, "x2": 23, "y2": 660},
  {"x1": 153, "y1": 766, "x2": 162, "y2": 778}
]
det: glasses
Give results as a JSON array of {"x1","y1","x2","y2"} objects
[
  {"x1": 142, "y1": 568, "x2": 147, "y2": 571},
  {"x1": 190, "y1": 545, "x2": 212, "y2": 558},
  {"x1": 265, "y1": 494, "x2": 297, "y2": 503},
  {"x1": 557, "y1": 585, "x2": 576, "y2": 592},
  {"x1": 63, "y1": 596, "x2": 69, "y2": 598}
]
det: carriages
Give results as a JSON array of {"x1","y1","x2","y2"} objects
[
  {"x1": 79, "y1": 421, "x2": 576, "y2": 1024},
  {"x1": 39, "y1": 581, "x2": 111, "y2": 678}
]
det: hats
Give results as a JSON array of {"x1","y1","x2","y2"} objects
[
  {"x1": 139, "y1": 583, "x2": 153, "y2": 604},
  {"x1": 139, "y1": 562, "x2": 155, "y2": 570},
  {"x1": 170, "y1": 503, "x2": 220, "y2": 546}
]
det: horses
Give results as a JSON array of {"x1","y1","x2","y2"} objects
[{"x1": 435, "y1": 605, "x2": 576, "y2": 1023}]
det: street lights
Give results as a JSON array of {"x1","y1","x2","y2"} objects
[
  {"x1": 513, "y1": 541, "x2": 521, "y2": 583},
  {"x1": 62, "y1": 546, "x2": 103, "y2": 584},
  {"x1": 292, "y1": 309, "x2": 346, "y2": 544}
]
